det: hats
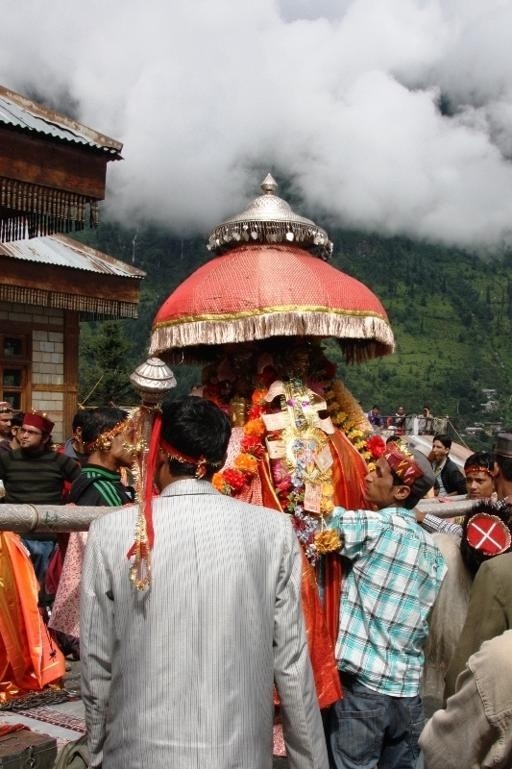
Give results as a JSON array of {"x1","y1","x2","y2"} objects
[
  {"x1": 495, "y1": 432, "x2": 512, "y2": 458},
  {"x1": 23, "y1": 408, "x2": 55, "y2": 435},
  {"x1": 383, "y1": 438, "x2": 434, "y2": 494},
  {"x1": 0, "y1": 401, "x2": 15, "y2": 413}
]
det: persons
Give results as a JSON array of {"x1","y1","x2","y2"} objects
[{"x1": 2, "y1": 395, "x2": 512, "y2": 768}]
